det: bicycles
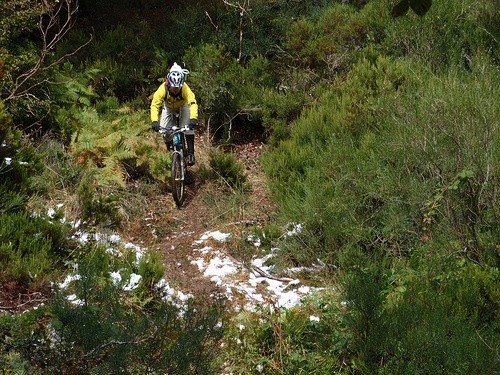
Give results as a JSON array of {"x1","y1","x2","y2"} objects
[{"x1": 157, "y1": 123, "x2": 193, "y2": 204}]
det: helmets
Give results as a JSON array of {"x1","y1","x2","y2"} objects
[{"x1": 166, "y1": 70, "x2": 184, "y2": 87}]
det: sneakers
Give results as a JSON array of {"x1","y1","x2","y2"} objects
[{"x1": 187, "y1": 152, "x2": 195, "y2": 165}]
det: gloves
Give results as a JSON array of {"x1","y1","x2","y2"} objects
[
  {"x1": 152, "y1": 122, "x2": 161, "y2": 132},
  {"x1": 189, "y1": 119, "x2": 198, "y2": 130}
]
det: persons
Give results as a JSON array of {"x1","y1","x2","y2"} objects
[{"x1": 150, "y1": 70, "x2": 199, "y2": 166}]
